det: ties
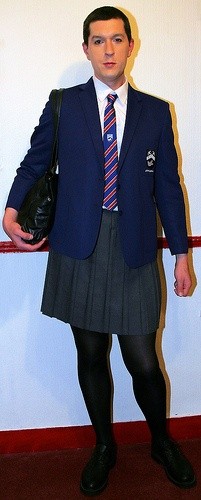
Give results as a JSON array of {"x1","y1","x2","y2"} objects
[{"x1": 104, "y1": 93, "x2": 120, "y2": 211}]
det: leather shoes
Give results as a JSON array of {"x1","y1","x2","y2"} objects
[
  {"x1": 79, "y1": 445, "x2": 116, "y2": 498},
  {"x1": 150, "y1": 440, "x2": 195, "y2": 488}
]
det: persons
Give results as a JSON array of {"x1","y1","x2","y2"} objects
[{"x1": 1, "y1": 6, "x2": 194, "y2": 498}]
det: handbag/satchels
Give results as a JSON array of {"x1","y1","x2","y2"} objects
[{"x1": 16, "y1": 89, "x2": 63, "y2": 246}]
